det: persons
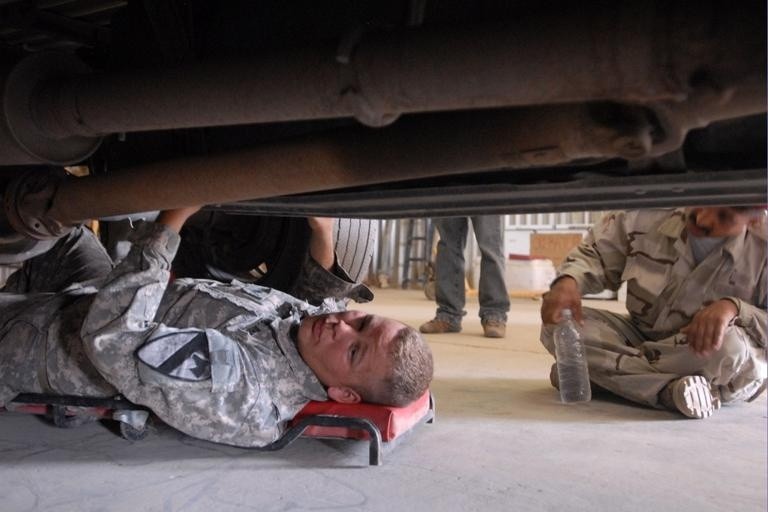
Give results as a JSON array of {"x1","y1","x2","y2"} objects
[
  {"x1": 419, "y1": 214, "x2": 511, "y2": 338},
  {"x1": 0, "y1": 206, "x2": 432, "y2": 448},
  {"x1": 540, "y1": 206, "x2": 768, "y2": 419}
]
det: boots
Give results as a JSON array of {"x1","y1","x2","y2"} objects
[
  {"x1": 420, "y1": 318, "x2": 506, "y2": 337},
  {"x1": 424, "y1": 261, "x2": 436, "y2": 300},
  {"x1": 551, "y1": 364, "x2": 604, "y2": 395},
  {"x1": 660, "y1": 376, "x2": 716, "y2": 418}
]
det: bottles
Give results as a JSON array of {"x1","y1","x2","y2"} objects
[{"x1": 552, "y1": 308, "x2": 591, "y2": 407}]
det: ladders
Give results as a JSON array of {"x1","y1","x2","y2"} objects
[{"x1": 402, "y1": 218, "x2": 434, "y2": 290}]
